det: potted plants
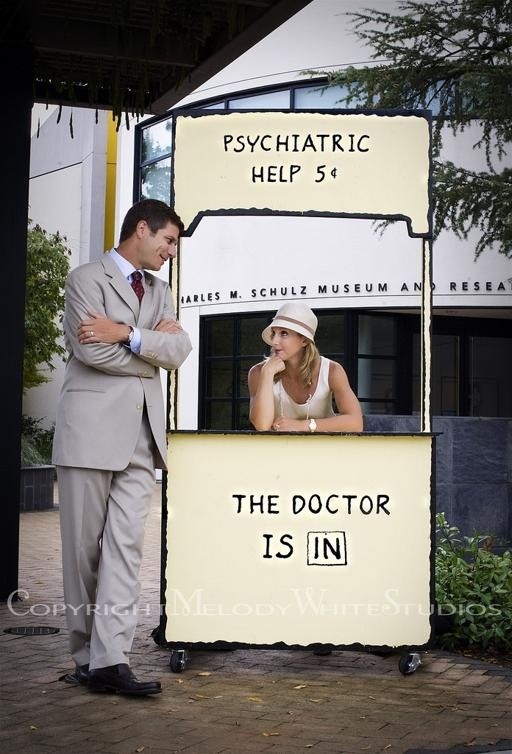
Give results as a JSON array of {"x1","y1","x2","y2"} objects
[{"x1": 20, "y1": 433, "x2": 54, "y2": 512}]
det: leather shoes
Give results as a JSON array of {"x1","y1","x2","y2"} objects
[{"x1": 74, "y1": 662, "x2": 163, "y2": 696}]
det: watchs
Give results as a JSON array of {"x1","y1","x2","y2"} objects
[
  {"x1": 308, "y1": 418, "x2": 317, "y2": 433},
  {"x1": 128, "y1": 326, "x2": 134, "y2": 343}
]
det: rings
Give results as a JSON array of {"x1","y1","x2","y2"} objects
[
  {"x1": 91, "y1": 330, "x2": 94, "y2": 336},
  {"x1": 276, "y1": 423, "x2": 280, "y2": 428}
]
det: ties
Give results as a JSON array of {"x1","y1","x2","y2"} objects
[{"x1": 130, "y1": 271, "x2": 145, "y2": 304}]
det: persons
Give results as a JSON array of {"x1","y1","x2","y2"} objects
[
  {"x1": 246, "y1": 300, "x2": 365, "y2": 432},
  {"x1": 50, "y1": 195, "x2": 193, "y2": 699}
]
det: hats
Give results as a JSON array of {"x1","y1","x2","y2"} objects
[{"x1": 262, "y1": 303, "x2": 319, "y2": 348}]
